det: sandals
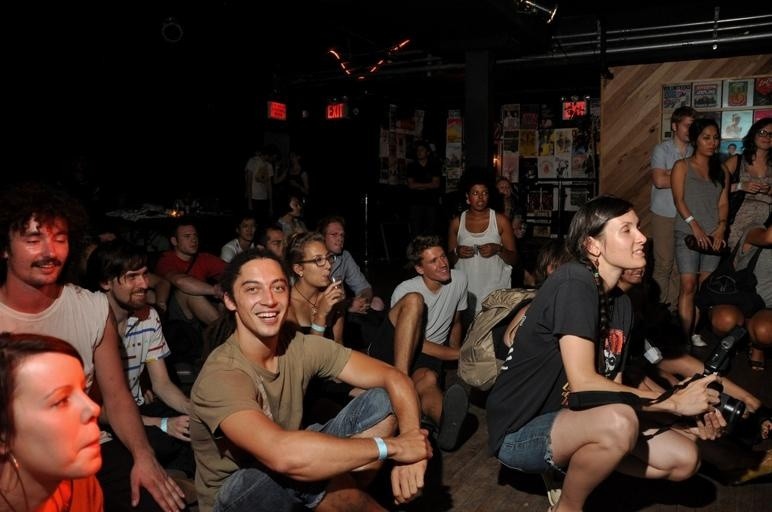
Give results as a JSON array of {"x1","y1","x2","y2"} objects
[{"x1": 749, "y1": 341, "x2": 765, "y2": 374}]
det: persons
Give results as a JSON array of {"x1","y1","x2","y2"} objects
[
  {"x1": 1, "y1": 149, "x2": 432, "y2": 512},
  {"x1": 390, "y1": 176, "x2": 772, "y2": 510},
  {"x1": 649, "y1": 109, "x2": 772, "y2": 369}
]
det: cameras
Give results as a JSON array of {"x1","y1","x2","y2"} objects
[{"x1": 683, "y1": 373, "x2": 746, "y2": 424}]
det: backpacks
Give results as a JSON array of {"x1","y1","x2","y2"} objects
[{"x1": 457, "y1": 287, "x2": 540, "y2": 391}]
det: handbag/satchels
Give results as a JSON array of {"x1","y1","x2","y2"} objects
[
  {"x1": 694, "y1": 265, "x2": 766, "y2": 319},
  {"x1": 727, "y1": 175, "x2": 745, "y2": 224}
]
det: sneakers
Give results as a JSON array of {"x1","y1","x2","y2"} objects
[
  {"x1": 692, "y1": 334, "x2": 707, "y2": 347},
  {"x1": 436, "y1": 385, "x2": 468, "y2": 452},
  {"x1": 541, "y1": 464, "x2": 562, "y2": 506}
]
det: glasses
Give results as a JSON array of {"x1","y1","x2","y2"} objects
[
  {"x1": 756, "y1": 131, "x2": 772, "y2": 138},
  {"x1": 299, "y1": 252, "x2": 337, "y2": 266}
]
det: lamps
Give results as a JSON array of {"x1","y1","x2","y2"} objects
[{"x1": 519, "y1": 0, "x2": 559, "y2": 28}]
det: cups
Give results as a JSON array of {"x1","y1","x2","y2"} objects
[{"x1": 329, "y1": 275, "x2": 346, "y2": 299}]
